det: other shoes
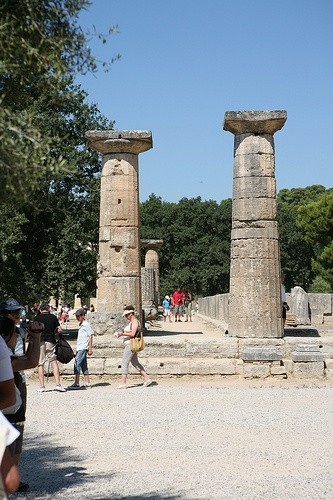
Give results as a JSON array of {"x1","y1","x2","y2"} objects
[
  {"x1": 68, "y1": 383, "x2": 79, "y2": 389},
  {"x1": 178, "y1": 319, "x2": 182, "y2": 322},
  {"x1": 143, "y1": 379, "x2": 152, "y2": 386},
  {"x1": 175, "y1": 320, "x2": 177, "y2": 322},
  {"x1": 115, "y1": 385, "x2": 126, "y2": 389},
  {"x1": 38, "y1": 386, "x2": 46, "y2": 392},
  {"x1": 16, "y1": 481, "x2": 29, "y2": 491},
  {"x1": 56, "y1": 386, "x2": 66, "y2": 392},
  {"x1": 82, "y1": 385, "x2": 91, "y2": 388}
]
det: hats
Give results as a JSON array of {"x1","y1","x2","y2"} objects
[
  {"x1": 0, "y1": 298, "x2": 25, "y2": 311},
  {"x1": 74, "y1": 310, "x2": 84, "y2": 316},
  {"x1": 122, "y1": 309, "x2": 134, "y2": 316},
  {"x1": 165, "y1": 295, "x2": 169, "y2": 299}
]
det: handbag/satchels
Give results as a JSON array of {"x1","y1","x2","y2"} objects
[
  {"x1": 131, "y1": 333, "x2": 144, "y2": 352},
  {"x1": 56, "y1": 335, "x2": 73, "y2": 363}
]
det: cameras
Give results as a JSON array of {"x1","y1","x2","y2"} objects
[{"x1": 20, "y1": 310, "x2": 40, "y2": 323}]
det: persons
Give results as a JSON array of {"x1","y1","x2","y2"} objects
[
  {"x1": 281, "y1": 270, "x2": 290, "y2": 327},
  {"x1": 0, "y1": 297, "x2": 95, "y2": 500},
  {"x1": 161, "y1": 286, "x2": 194, "y2": 322},
  {"x1": 113, "y1": 305, "x2": 153, "y2": 389}
]
